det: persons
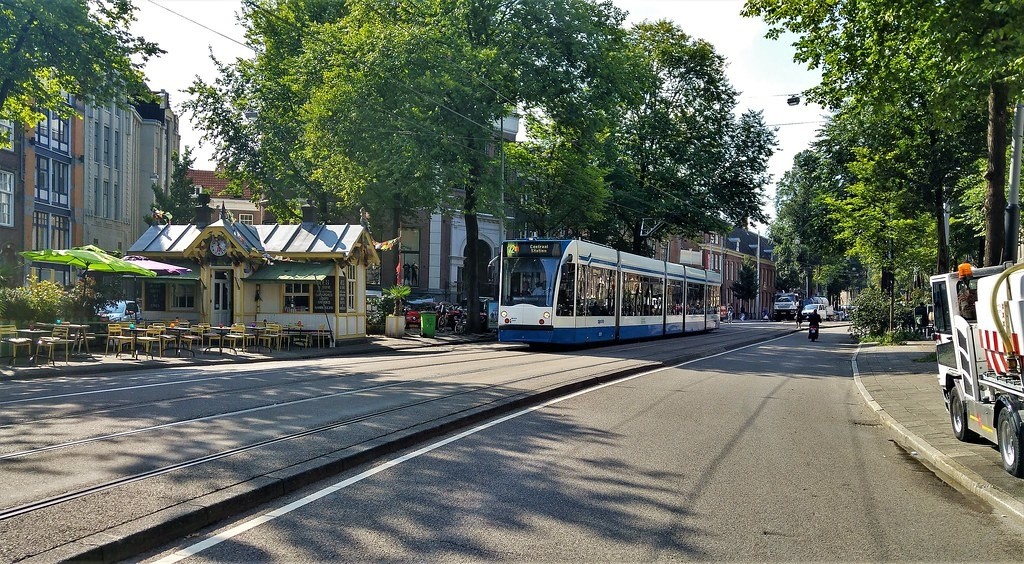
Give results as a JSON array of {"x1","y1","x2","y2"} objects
[
  {"x1": 739, "y1": 304, "x2": 745, "y2": 322},
  {"x1": 807, "y1": 309, "x2": 822, "y2": 335},
  {"x1": 794, "y1": 306, "x2": 803, "y2": 330},
  {"x1": 761, "y1": 306, "x2": 768, "y2": 321},
  {"x1": 727, "y1": 303, "x2": 734, "y2": 325},
  {"x1": 518, "y1": 280, "x2": 553, "y2": 296},
  {"x1": 915, "y1": 311, "x2": 930, "y2": 337}
]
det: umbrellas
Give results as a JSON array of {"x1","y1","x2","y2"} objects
[
  {"x1": 17, "y1": 244, "x2": 157, "y2": 344},
  {"x1": 85, "y1": 254, "x2": 192, "y2": 361}
]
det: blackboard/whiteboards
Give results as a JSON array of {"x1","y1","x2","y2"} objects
[
  {"x1": 339, "y1": 275, "x2": 347, "y2": 313},
  {"x1": 144, "y1": 282, "x2": 166, "y2": 312},
  {"x1": 313, "y1": 275, "x2": 335, "y2": 314}
]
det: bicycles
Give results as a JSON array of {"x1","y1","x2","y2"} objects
[
  {"x1": 435, "y1": 306, "x2": 468, "y2": 334},
  {"x1": 727, "y1": 308, "x2": 734, "y2": 323}
]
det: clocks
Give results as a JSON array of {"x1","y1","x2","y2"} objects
[{"x1": 210, "y1": 238, "x2": 227, "y2": 256}]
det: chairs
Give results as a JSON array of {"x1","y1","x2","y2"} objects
[{"x1": 0, "y1": 321, "x2": 333, "y2": 364}]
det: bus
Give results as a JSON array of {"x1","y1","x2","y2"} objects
[{"x1": 488, "y1": 217, "x2": 721, "y2": 352}]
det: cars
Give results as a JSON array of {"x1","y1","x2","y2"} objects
[
  {"x1": 405, "y1": 302, "x2": 446, "y2": 328},
  {"x1": 801, "y1": 304, "x2": 826, "y2": 321},
  {"x1": 448, "y1": 303, "x2": 487, "y2": 324},
  {"x1": 94, "y1": 300, "x2": 141, "y2": 323}
]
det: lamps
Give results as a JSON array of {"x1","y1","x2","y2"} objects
[
  {"x1": 29, "y1": 137, "x2": 37, "y2": 146},
  {"x1": 79, "y1": 155, "x2": 84, "y2": 164}
]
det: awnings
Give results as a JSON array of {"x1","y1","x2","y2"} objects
[
  {"x1": 122, "y1": 256, "x2": 208, "y2": 289},
  {"x1": 239, "y1": 257, "x2": 336, "y2": 285}
]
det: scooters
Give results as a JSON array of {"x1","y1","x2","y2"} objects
[{"x1": 806, "y1": 318, "x2": 823, "y2": 341}]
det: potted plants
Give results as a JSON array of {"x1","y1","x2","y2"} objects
[{"x1": 383, "y1": 285, "x2": 411, "y2": 337}]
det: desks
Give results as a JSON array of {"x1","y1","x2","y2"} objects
[
  {"x1": 161, "y1": 327, "x2": 195, "y2": 358},
  {"x1": 10, "y1": 329, "x2": 55, "y2": 366},
  {"x1": 241, "y1": 327, "x2": 274, "y2": 354},
  {"x1": 29, "y1": 324, "x2": 95, "y2": 361},
  {"x1": 115, "y1": 328, "x2": 154, "y2": 360},
  {"x1": 204, "y1": 327, "x2": 237, "y2": 357}
]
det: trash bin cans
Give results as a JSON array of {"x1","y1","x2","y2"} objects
[{"x1": 420, "y1": 311, "x2": 437, "y2": 336}]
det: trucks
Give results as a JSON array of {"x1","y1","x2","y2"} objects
[
  {"x1": 773, "y1": 293, "x2": 800, "y2": 321},
  {"x1": 914, "y1": 261, "x2": 1024, "y2": 478}
]
